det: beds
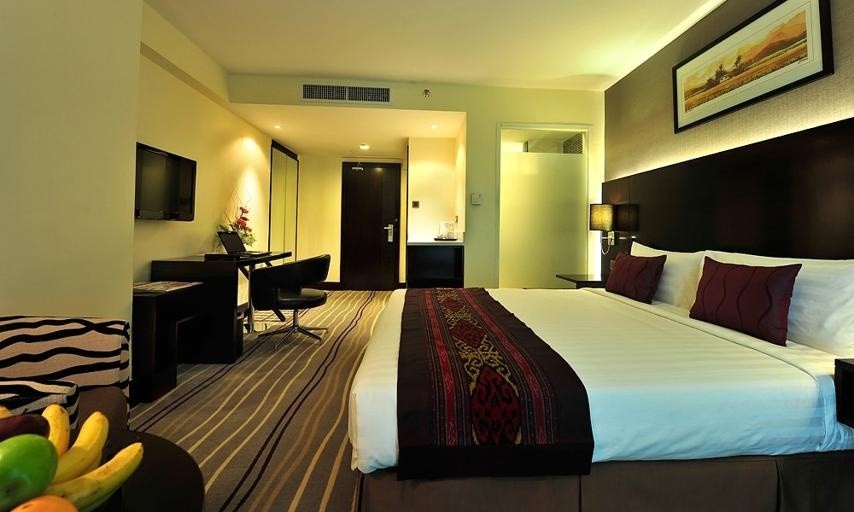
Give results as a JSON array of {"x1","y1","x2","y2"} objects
[{"x1": 348, "y1": 286, "x2": 853, "y2": 511}]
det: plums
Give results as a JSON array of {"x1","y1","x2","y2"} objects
[{"x1": 0, "y1": 413, "x2": 50, "y2": 441}]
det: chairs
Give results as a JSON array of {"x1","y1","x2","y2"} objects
[
  {"x1": 0, "y1": 314, "x2": 131, "y2": 427},
  {"x1": 250, "y1": 255, "x2": 330, "y2": 351}
]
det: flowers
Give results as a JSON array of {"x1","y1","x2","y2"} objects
[{"x1": 215, "y1": 207, "x2": 256, "y2": 252}]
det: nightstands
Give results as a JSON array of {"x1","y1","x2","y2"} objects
[
  {"x1": 834, "y1": 358, "x2": 853, "y2": 429},
  {"x1": 556, "y1": 273, "x2": 601, "y2": 288}
]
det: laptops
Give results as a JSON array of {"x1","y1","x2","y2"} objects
[{"x1": 217, "y1": 231, "x2": 272, "y2": 257}]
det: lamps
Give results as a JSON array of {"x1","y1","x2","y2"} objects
[{"x1": 590, "y1": 204, "x2": 615, "y2": 245}]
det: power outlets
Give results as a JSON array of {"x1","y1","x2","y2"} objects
[{"x1": 607, "y1": 231, "x2": 614, "y2": 246}]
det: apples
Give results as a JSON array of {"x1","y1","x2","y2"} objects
[{"x1": 0, "y1": 433, "x2": 57, "y2": 510}]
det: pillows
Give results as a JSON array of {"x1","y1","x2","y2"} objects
[
  {"x1": 604, "y1": 250, "x2": 667, "y2": 303},
  {"x1": 690, "y1": 249, "x2": 853, "y2": 356},
  {"x1": 689, "y1": 256, "x2": 802, "y2": 347},
  {"x1": 630, "y1": 241, "x2": 705, "y2": 310}
]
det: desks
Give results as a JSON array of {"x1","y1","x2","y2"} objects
[
  {"x1": 0, "y1": 429, "x2": 206, "y2": 512},
  {"x1": 405, "y1": 241, "x2": 464, "y2": 288},
  {"x1": 151, "y1": 250, "x2": 292, "y2": 364}
]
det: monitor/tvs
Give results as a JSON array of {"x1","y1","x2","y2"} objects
[{"x1": 137, "y1": 141, "x2": 196, "y2": 222}]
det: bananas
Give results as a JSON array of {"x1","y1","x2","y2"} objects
[{"x1": 42, "y1": 401, "x2": 144, "y2": 512}]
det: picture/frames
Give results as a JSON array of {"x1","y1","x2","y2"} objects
[{"x1": 672, "y1": 0, "x2": 835, "y2": 133}]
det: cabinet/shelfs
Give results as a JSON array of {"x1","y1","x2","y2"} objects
[{"x1": 133, "y1": 280, "x2": 204, "y2": 403}]
